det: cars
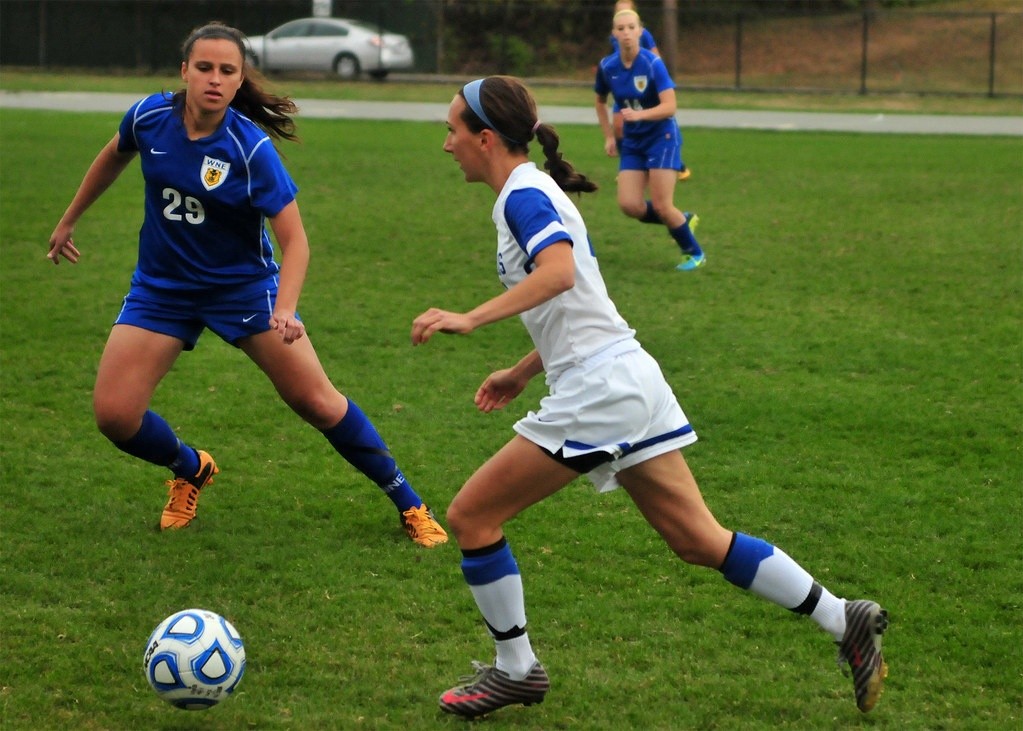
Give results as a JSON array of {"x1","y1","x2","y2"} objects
[{"x1": 239, "y1": 15, "x2": 416, "y2": 81}]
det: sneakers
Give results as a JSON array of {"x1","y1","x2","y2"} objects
[
  {"x1": 834, "y1": 599, "x2": 890, "y2": 712},
  {"x1": 670, "y1": 212, "x2": 699, "y2": 245},
  {"x1": 160, "y1": 449, "x2": 219, "y2": 534},
  {"x1": 674, "y1": 251, "x2": 704, "y2": 271},
  {"x1": 677, "y1": 168, "x2": 690, "y2": 180},
  {"x1": 400, "y1": 501, "x2": 449, "y2": 550},
  {"x1": 438, "y1": 656, "x2": 549, "y2": 720}
]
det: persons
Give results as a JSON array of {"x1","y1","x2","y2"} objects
[
  {"x1": 595, "y1": 10, "x2": 705, "y2": 270},
  {"x1": 410, "y1": 76, "x2": 890, "y2": 716},
  {"x1": 611, "y1": 1, "x2": 690, "y2": 179},
  {"x1": 48, "y1": 23, "x2": 451, "y2": 549}
]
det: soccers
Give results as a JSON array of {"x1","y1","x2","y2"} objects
[{"x1": 143, "y1": 608, "x2": 246, "y2": 709}]
